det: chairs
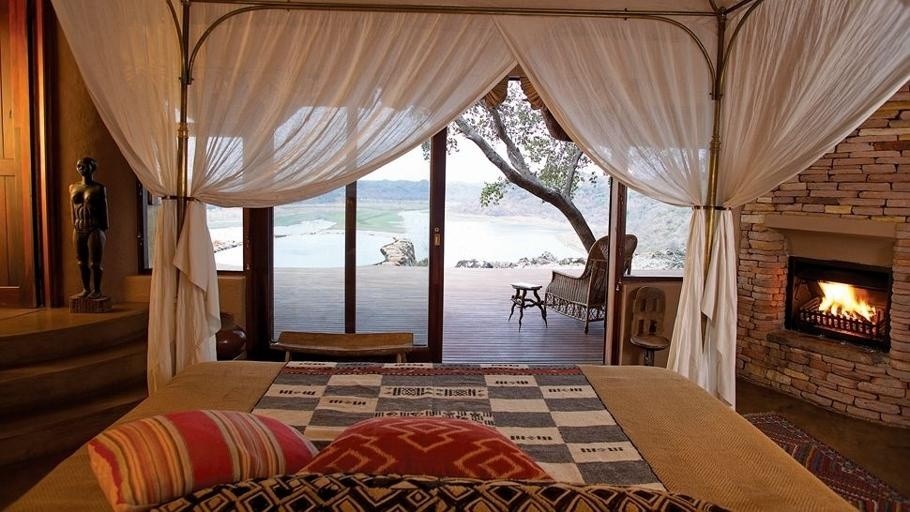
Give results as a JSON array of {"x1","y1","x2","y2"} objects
[
  {"x1": 544, "y1": 234, "x2": 638, "y2": 335},
  {"x1": 630, "y1": 286, "x2": 670, "y2": 366}
]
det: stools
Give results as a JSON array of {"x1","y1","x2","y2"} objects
[{"x1": 508, "y1": 282, "x2": 549, "y2": 332}]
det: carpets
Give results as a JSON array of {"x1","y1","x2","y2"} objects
[{"x1": 741, "y1": 412, "x2": 909, "y2": 512}]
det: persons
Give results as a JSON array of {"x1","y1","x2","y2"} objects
[{"x1": 68, "y1": 156, "x2": 110, "y2": 299}]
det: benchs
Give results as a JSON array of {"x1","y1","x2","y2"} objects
[{"x1": 271, "y1": 331, "x2": 415, "y2": 362}]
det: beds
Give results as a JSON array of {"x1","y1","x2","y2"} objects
[{"x1": 1, "y1": 361, "x2": 862, "y2": 512}]
